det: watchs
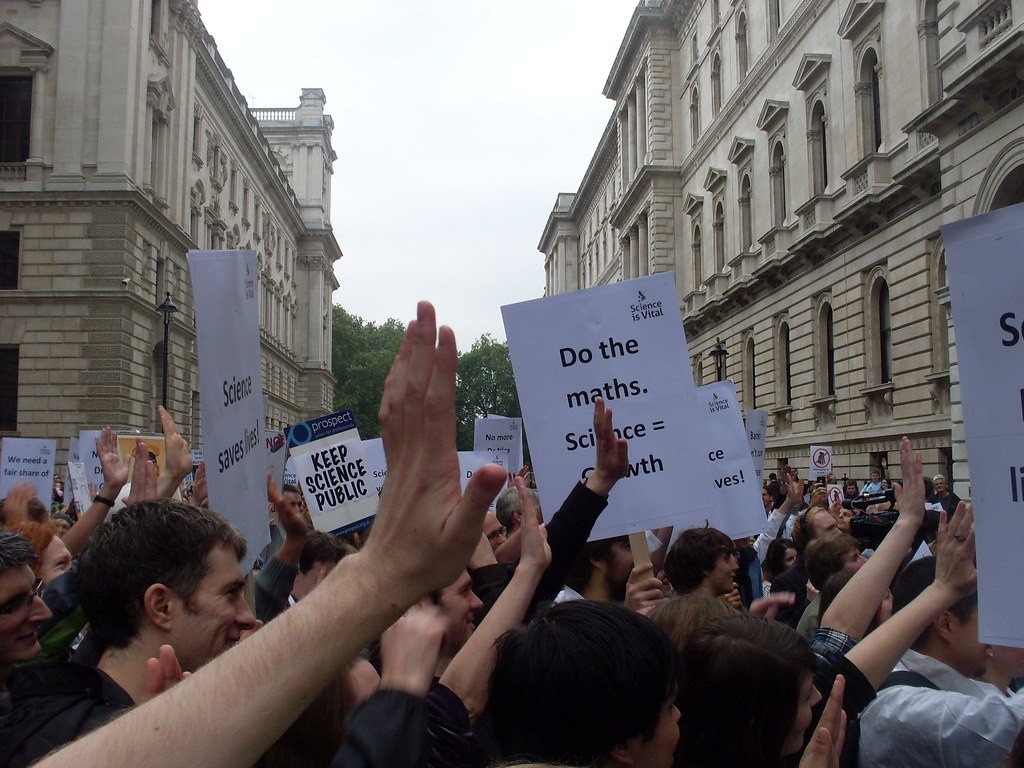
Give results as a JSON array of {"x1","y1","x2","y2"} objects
[{"x1": 92, "y1": 491, "x2": 117, "y2": 507}]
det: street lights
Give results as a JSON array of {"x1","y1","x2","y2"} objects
[{"x1": 154, "y1": 288, "x2": 179, "y2": 432}]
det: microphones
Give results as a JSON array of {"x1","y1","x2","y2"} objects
[{"x1": 864, "y1": 476, "x2": 934, "y2": 499}]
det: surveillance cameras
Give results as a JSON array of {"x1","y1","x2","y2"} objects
[{"x1": 122, "y1": 278, "x2": 130, "y2": 286}]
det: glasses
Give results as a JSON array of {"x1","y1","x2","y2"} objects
[
  {"x1": 0, "y1": 577, "x2": 46, "y2": 615},
  {"x1": 487, "y1": 527, "x2": 507, "y2": 541}
]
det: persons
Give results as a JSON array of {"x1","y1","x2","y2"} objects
[{"x1": 0, "y1": 291, "x2": 1023, "y2": 768}]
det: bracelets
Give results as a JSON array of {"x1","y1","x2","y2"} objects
[{"x1": 581, "y1": 465, "x2": 610, "y2": 500}]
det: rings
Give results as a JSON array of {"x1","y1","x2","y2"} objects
[{"x1": 954, "y1": 534, "x2": 967, "y2": 542}]
